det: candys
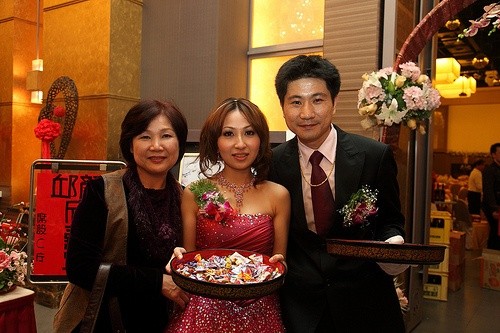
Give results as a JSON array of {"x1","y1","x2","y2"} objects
[{"x1": 174, "y1": 252, "x2": 281, "y2": 284}]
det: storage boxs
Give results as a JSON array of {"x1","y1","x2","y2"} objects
[
  {"x1": 423, "y1": 211, "x2": 467, "y2": 301},
  {"x1": 474, "y1": 248, "x2": 500, "y2": 291}
]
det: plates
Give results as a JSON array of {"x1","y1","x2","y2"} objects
[
  {"x1": 170, "y1": 249, "x2": 287, "y2": 300},
  {"x1": 326, "y1": 239, "x2": 447, "y2": 266}
]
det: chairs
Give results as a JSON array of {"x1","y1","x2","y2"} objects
[{"x1": 429, "y1": 172, "x2": 500, "y2": 249}]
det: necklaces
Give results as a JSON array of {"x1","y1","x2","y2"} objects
[
  {"x1": 296, "y1": 152, "x2": 335, "y2": 188},
  {"x1": 217, "y1": 170, "x2": 256, "y2": 212}
]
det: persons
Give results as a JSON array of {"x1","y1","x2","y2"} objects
[
  {"x1": 481, "y1": 142, "x2": 500, "y2": 250},
  {"x1": 265, "y1": 55, "x2": 410, "y2": 333},
  {"x1": 166, "y1": 97, "x2": 291, "y2": 333},
  {"x1": 65, "y1": 98, "x2": 191, "y2": 333},
  {"x1": 467, "y1": 159, "x2": 485, "y2": 215}
]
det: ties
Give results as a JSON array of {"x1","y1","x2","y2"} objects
[{"x1": 309, "y1": 151, "x2": 335, "y2": 239}]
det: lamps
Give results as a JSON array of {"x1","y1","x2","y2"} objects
[{"x1": 436, "y1": 57, "x2": 477, "y2": 98}]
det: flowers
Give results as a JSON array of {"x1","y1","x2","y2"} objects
[
  {"x1": 393, "y1": 276, "x2": 408, "y2": 316},
  {"x1": 0, "y1": 212, "x2": 28, "y2": 295},
  {"x1": 34, "y1": 105, "x2": 66, "y2": 159},
  {"x1": 456, "y1": 1, "x2": 500, "y2": 43},
  {"x1": 336, "y1": 184, "x2": 381, "y2": 227},
  {"x1": 357, "y1": 61, "x2": 441, "y2": 135},
  {"x1": 189, "y1": 180, "x2": 234, "y2": 227}
]
